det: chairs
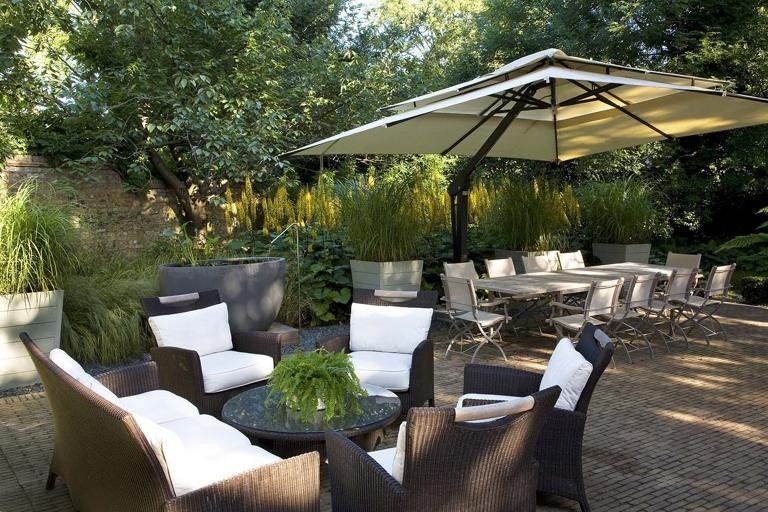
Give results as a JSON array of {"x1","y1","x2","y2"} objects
[
  {"x1": 137, "y1": 287, "x2": 282, "y2": 419},
  {"x1": 441, "y1": 243, "x2": 737, "y2": 368},
  {"x1": 314, "y1": 286, "x2": 439, "y2": 416},
  {"x1": 315, "y1": 382, "x2": 563, "y2": 511},
  {"x1": 459, "y1": 315, "x2": 616, "y2": 512}
]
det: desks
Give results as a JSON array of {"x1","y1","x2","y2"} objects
[{"x1": 223, "y1": 376, "x2": 400, "y2": 463}]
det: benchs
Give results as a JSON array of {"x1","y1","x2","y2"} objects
[{"x1": 14, "y1": 327, "x2": 320, "y2": 511}]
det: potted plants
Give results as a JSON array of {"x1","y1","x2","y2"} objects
[
  {"x1": 335, "y1": 172, "x2": 429, "y2": 302},
  {"x1": 153, "y1": 217, "x2": 286, "y2": 330},
  {"x1": 262, "y1": 343, "x2": 364, "y2": 424},
  {"x1": 0, "y1": 173, "x2": 74, "y2": 389},
  {"x1": 572, "y1": 176, "x2": 668, "y2": 262}
]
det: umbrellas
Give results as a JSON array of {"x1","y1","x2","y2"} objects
[{"x1": 271, "y1": 47, "x2": 768, "y2": 305}]
